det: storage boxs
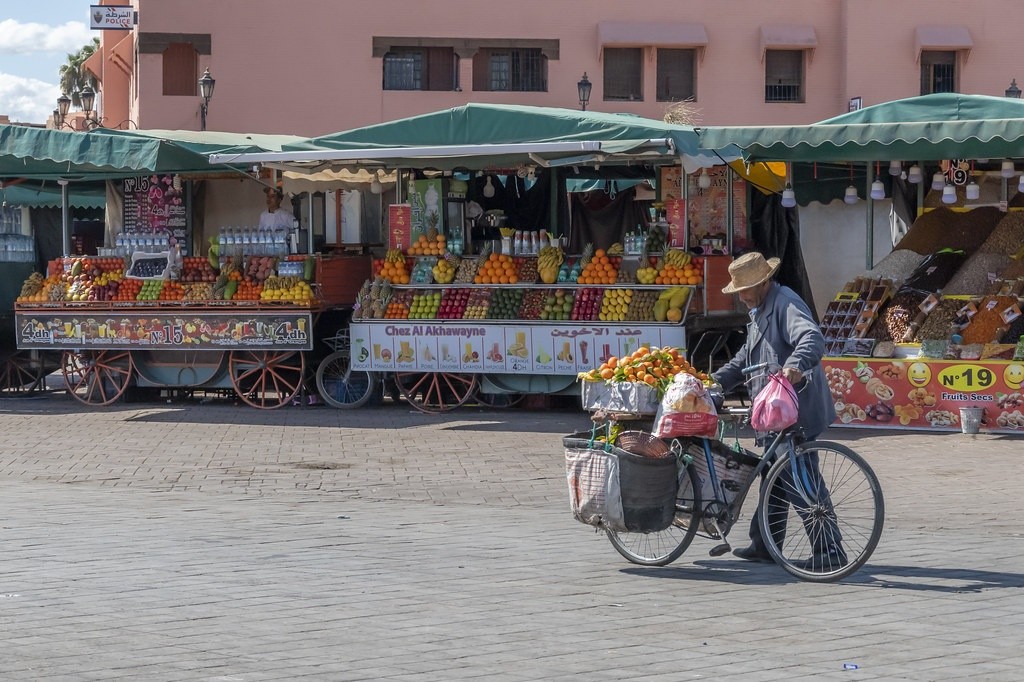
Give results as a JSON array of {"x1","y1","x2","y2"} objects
[
  {"x1": 582, "y1": 377, "x2": 658, "y2": 414},
  {"x1": 126, "y1": 250, "x2": 176, "y2": 280}
]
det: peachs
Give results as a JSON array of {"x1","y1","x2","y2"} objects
[{"x1": 559, "y1": 351, "x2": 574, "y2": 362}]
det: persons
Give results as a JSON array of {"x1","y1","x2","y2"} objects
[
  {"x1": 709, "y1": 252, "x2": 848, "y2": 569},
  {"x1": 259, "y1": 186, "x2": 295, "y2": 230}
]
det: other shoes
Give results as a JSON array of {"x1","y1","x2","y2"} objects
[
  {"x1": 796, "y1": 544, "x2": 847, "y2": 571},
  {"x1": 732, "y1": 542, "x2": 784, "y2": 562}
]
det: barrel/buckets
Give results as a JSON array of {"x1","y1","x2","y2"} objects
[{"x1": 959, "y1": 408, "x2": 983, "y2": 434}]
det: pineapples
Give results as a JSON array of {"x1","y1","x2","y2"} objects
[
  {"x1": 640, "y1": 252, "x2": 649, "y2": 268},
  {"x1": 207, "y1": 276, "x2": 225, "y2": 300},
  {"x1": 425, "y1": 209, "x2": 492, "y2": 266},
  {"x1": 355, "y1": 280, "x2": 391, "y2": 319},
  {"x1": 580, "y1": 243, "x2": 593, "y2": 267}
]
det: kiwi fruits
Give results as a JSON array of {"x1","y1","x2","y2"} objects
[{"x1": 627, "y1": 291, "x2": 659, "y2": 320}]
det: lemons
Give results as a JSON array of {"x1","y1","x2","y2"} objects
[{"x1": 260, "y1": 281, "x2": 314, "y2": 299}]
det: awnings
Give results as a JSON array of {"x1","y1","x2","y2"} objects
[
  {"x1": 597, "y1": 20, "x2": 709, "y2": 63},
  {"x1": 759, "y1": 24, "x2": 818, "y2": 63},
  {"x1": 109, "y1": 32, "x2": 133, "y2": 77},
  {"x1": 80, "y1": 47, "x2": 103, "y2": 82},
  {"x1": 915, "y1": 25, "x2": 973, "y2": 64}
]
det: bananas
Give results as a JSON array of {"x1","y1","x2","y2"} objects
[
  {"x1": 385, "y1": 248, "x2": 406, "y2": 264},
  {"x1": 537, "y1": 246, "x2": 563, "y2": 273},
  {"x1": 666, "y1": 248, "x2": 691, "y2": 268},
  {"x1": 607, "y1": 243, "x2": 623, "y2": 254}
]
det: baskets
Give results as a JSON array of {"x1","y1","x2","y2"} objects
[{"x1": 620, "y1": 430, "x2": 670, "y2": 459}]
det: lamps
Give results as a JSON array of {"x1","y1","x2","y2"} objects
[
  {"x1": 53, "y1": 81, "x2": 138, "y2": 132},
  {"x1": 198, "y1": 67, "x2": 216, "y2": 131},
  {"x1": 1004, "y1": 79, "x2": 1022, "y2": 98},
  {"x1": 577, "y1": 71, "x2": 592, "y2": 111}
]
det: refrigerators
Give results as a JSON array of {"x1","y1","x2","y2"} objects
[{"x1": 408, "y1": 178, "x2": 468, "y2": 250}]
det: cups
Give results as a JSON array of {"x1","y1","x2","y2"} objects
[
  {"x1": 374, "y1": 332, "x2": 629, "y2": 363},
  {"x1": 514, "y1": 229, "x2": 548, "y2": 254},
  {"x1": 75, "y1": 325, "x2": 81, "y2": 338},
  {"x1": 64, "y1": 323, "x2": 71, "y2": 333},
  {"x1": 99, "y1": 326, "x2": 104, "y2": 337},
  {"x1": 236, "y1": 323, "x2": 304, "y2": 335}
]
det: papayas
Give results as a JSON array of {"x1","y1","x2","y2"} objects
[{"x1": 653, "y1": 286, "x2": 690, "y2": 321}]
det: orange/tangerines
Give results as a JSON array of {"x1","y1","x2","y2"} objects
[
  {"x1": 577, "y1": 249, "x2": 617, "y2": 284},
  {"x1": 379, "y1": 235, "x2": 446, "y2": 284},
  {"x1": 475, "y1": 253, "x2": 518, "y2": 284},
  {"x1": 509, "y1": 343, "x2": 529, "y2": 358},
  {"x1": 655, "y1": 257, "x2": 704, "y2": 285}
]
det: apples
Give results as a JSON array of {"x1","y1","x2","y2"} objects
[
  {"x1": 227, "y1": 271, "x2": 263, "y2": 298},
  {"x1": 589, "y1": 347, "x2": 712, "y2": 403},
  {"x1": 16, "y1": 258, "x2": 216, "y2": 300},
  {"x1": 636, "y1": 267, "x2": 658, "y2": 284},
  {"x1": 572, "y1": 289, "x2": 603, "y2": 321},
  {"x1": 374, "y1": 259, "x2": 470, "y2": 319},
  {"x1": 610, "y1": 258, "x2": 622, "y2": 270},
  {"x1": 650, "y1": 257, "x2": 657, "y2": 264}
]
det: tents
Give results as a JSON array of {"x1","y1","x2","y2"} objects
[
  {"x1": 282, "y1": 103, "x2": 733, "y2": 256},
  {"x1": 699, "y1": 94, "x2": 1024, "y2": 269},
  {"x1": 0, "y1": 124, "x2": 313, "y2": 256}
]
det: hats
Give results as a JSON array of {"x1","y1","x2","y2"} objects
[
  {"x1": 263, "y1": 186, "x2": 282, "y2": 195},
  {"x1": 721, "y1": 252, "x2": 781, "y2": 294}
]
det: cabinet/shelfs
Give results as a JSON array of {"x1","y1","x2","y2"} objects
[{"x1": 351, "y1": 256, "x2": 697, "y2": 326}]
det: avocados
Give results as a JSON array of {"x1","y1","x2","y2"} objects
[{"x1": 486, "y1": 289, "x2": 521, "y2": 319}]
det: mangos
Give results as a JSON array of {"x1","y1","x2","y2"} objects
[
  {"x1": 599, "y1": 289, "x2": 633, "y2": 321},
  {"x1": 540, "y1": 289, "x2": 574, "y2": 320}
]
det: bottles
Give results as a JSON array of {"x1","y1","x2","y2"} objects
[
  {"x1": 265, "y1": 227, "x2": 274, "y2": 257},
  {"x1": 274, "y1": 226, "x2": 285, "y2": 258},
  {"x1": 146, "y1": 232, "x2": 153, "y2": 253},
  {"x1": 624, "y1": 233, "x2": 630, "y2": 251},
  {"x1": 446, "y1": 226, "x2": 462, "y2": 255},
  {"x1": 425, "y1": 182, "x2": 439, "y2": 231},
  {"x1": 242, "y1": 226, "x2": 250, "y2": 256},
  {"x1": 161, "y1": 230, "x2": 169, "y2": 251},
  {"x1": 116, "y1": 233, "x2": 146, "y2": 256},
  {"x1": 219, "y1": 226, "x2": 226, "y2": 255},
  {"x1": 259, "y1": 227, "x2": 265, "y2": 257},
  {"x1": 153, "y1": 231, "x2": 162, "y2": 252},
  {"x1": 250, "y1": 227, "x2": 259, "y2": 256},
  {"x1": 642, "y1": 231, "x2": 647, "y2": 249},
  {"x1": 234, "y1": 226, "x2": 242, "y2": 256},
  {"x1": 635, "y1": 224, "x2": 642, "y2": 251},
  {"x1": 278, "y1": 261, "x2": 304, "y2": 276},
  {"x1": 226, "y1": 227, "x2": 234, "y2": 256},
  {"x1": 630, "y1": 232, "x2": 635, "y2": 251}
]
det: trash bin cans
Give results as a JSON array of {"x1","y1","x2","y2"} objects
[{"x1": 959, "y1": 408, "x2": 984, "y2": 434}]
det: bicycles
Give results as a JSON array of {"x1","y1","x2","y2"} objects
[
  {"x1": 316, "y1": 329, "x2": 392, "y2": 410},
  {"x1": 591, "y1": 363, "x2": 885, "y2": 583}
]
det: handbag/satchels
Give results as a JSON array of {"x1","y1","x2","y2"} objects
[{"x1": 751, "y1": 374, "x2": 799, "y2": 430}]
